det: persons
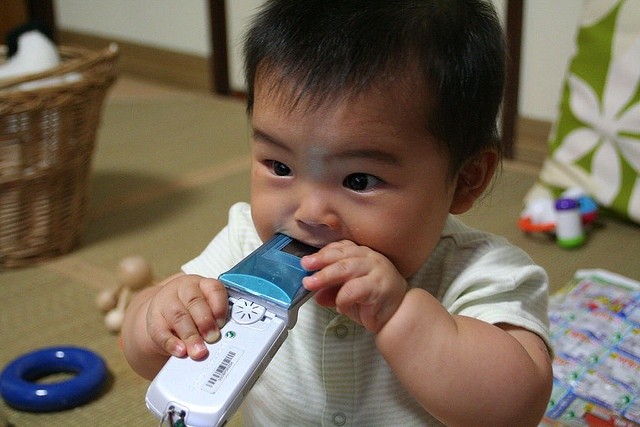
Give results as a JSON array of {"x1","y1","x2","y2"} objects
[{"x1": 120, "y1": 0, "x2": 552, "y2": 425}]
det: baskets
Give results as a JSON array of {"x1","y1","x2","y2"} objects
[{"x1": 0, "y1": 43, "x2": 118, "y2": 269}]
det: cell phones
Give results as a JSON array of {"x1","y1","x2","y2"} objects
[{"x1": 144, "y1": 234, "x2": 328, "y2": 426}]
